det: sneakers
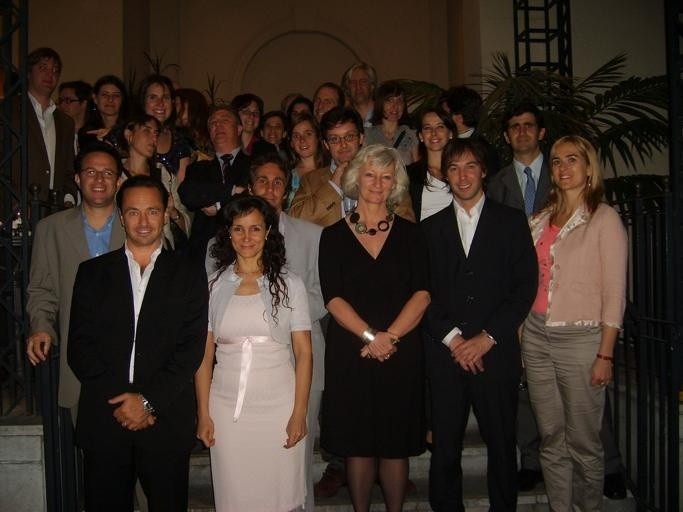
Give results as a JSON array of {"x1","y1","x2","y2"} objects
[
  {"x1": 314, "y1": 460, "x2": 346, "y2": 497},
  {"x1": 403, "y1": 478, "x2": 420, "y2": 496}
]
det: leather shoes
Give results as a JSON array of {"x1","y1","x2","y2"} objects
[
  {"x1": 514, "y1": 465, "x2": 546, "y2": 492},
  {"x1": 602, "y1": 472, "x2": 628, "y2": 500}
]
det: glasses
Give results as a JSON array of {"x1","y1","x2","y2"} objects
[
  {"x1": 327, "y1": 134, "x2": 355, "y2": 144},
  {"x1": 343, "y1": 79, "x2": 370, "y2": 87},
  {"x1": 57, "y1": 98, "x2": 82, "y2": 104}
]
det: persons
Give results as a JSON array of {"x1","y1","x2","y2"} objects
[{"x1": 0, "y1": 48, "x2": 630, "y2": 511}]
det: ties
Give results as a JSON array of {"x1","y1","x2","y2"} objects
[
  {"x1": 219, "y1": 153, "x2": 233, "y2": 183},
  {"x1": 522, "y1": 166, "x2": 536, "y2": 215}
]
